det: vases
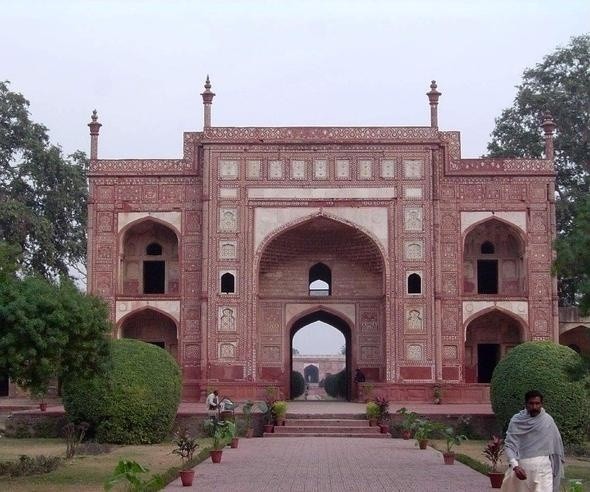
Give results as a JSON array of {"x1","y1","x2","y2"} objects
[{"x1": 57, "y1": 398, "x2": 64, "y2": 404}]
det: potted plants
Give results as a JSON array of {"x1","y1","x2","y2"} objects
[
  {"x1": 168, "y1": 386, "x2": 288, "y2": 486},
  {"x1": 28, "y1": 387, "x2": 48, "y2": 411},
  {"x1": 362, "y1": 382, "x2": 505, "y2": 488}
]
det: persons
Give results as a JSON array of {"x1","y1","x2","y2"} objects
[
  {"x1": 353, "y1": 368, "x2": 365, "y2": 383},
  {"x1": 205, "y1": 390, "x2": 221, "y2": 410},
  {"x1": 499, "y1": 389, "x2": 566, "y2": 491}
]
